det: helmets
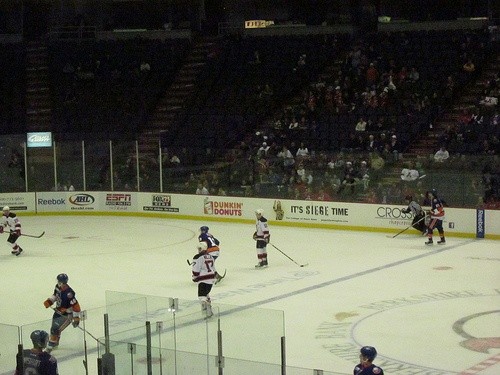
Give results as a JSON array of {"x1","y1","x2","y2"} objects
[
  {"x1": 428, "y1": 188, "x2": 437, "y2": 196},
  {"x1": 197, "y1": 241, "x2": 208, "y2": 251},
  {"x1": 361, "y1": 346, "x2": 378, "y2": 361},
  {"x1": 3, "y1": 206, "x2": 10, "y2": 211},
  {"x1": 347, "y1": 160, "x2": 367, "y2": 165},
  {"x1": 255, "y1": 208, "x2": 265, "y2": 217},
  {"x1": 405, "y1": 195, "x2": 413, "y2": 201},
  {"x1": 57, "y1": 274, "x2": 68, "y2": 282},
  {"x1": 200, "y1": 226, "x2": 209, "y2": 230},
  {"x1": 30, "y1": 330, "x2": 48, "y2": 344}
]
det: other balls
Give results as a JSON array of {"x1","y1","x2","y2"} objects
[{"x1": 3, "y1": 230, "x2": 45, "y2": 238}]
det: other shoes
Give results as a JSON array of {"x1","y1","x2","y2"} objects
[
  {"x1": 203, "y1": 311, "x2": 213, "y2": 319},
  {"x1": 202, "y1": 303, "x2": 207, "y2": 310}
]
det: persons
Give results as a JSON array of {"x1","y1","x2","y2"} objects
[
  {"x1": 44, "y1": 274, "x2": 81, "y2": 354},
  {"x1": 252, "y1": 209, "x2": 271, "y2": 268},
  {"x1": 354, "y1": 346, "x2": 384, "y2": 375},
  {"x1": 0, "y1": 145, "x2": 156, "y2": 192},
  {"x1": 156, "y1": 28, "x2": 500, "y2": 209},
  {"x1": 140, "y1": 60, "x2": 151, "y2": 72},
  {"x1": 14, "y1": 330, "x2": 58, "y2": 375},
  {"x1": 192, "y1": 242, "x2": 216, "y2": 320},
  {"x1": 402, "y1": 195, "x2": 429, "y2": 235},
  {"x1": 425, "y1": 190, "x2": 446, "y2": 245},
  {"x1": 200, "y1": 226, "x2": 220, "y2": 246},
  {"x1": 0, "y1": 206, "x2": 24, "y2": 257},
  {"x1": 197, "y1": 233, "x2": 222, "y2": 284}
]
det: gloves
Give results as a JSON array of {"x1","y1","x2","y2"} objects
[
  {"x1": 44, "y1": 299, "x2": 54, "y2": 308},
  {"x1": 265, "y1": 237, "x2": 269, "y2": 244},
  {"x1": 253, "y1": 232, "x2": 257, "y2": 240},
  {"x1": 0, "y1": 226, "x2": 3, "y2": 233},
  {"x1": 402, "y1": 210, "x2": 406, "y2": 214},
  {"x1": 427, "y1": 210, "x2": 435, "y2": 218},
  {"x1": 10, "y1": 231, "x2": 17, "y2": 238},
  {"x1": 72, "y1": 317, "x2": 80, "y2": 328}
]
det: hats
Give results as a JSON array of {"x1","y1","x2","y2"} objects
[
  {"x1": 256, "y1": 132, "x2": 268, "y2": 145},
  {"x1": 369, "y1": 133, "x2": 396, "y2": 139}
]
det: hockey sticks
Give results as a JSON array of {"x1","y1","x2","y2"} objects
[
  {"x1": 186, "y1": 259, "x2": 193, "y2": 266},
  {"x1": 385, "y1": 214, "x2": 431, "y2": 239},
  {"x1": 49, "y1": 305, "x2": 105, "y2": 346},
  {"x1": 268, "y1": 242, "x2": 309, "y2": 268},
  {"x1": 215, "y1": 268, "x2": 227, "y2": 280}
]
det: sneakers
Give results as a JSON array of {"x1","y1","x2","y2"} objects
[
  {"x1": 423, "y1": 231, "x2": 428, "y2": 236},
  {"x1": 255, "y1": 261, "x2": 268, "y2": 268},
  {"x1": 215, "y1": 274, "x2": 224, "y2": 285},
  {"x1": 43, "y1": 340, "x2": 59, "y2": 353},
  {"x1": 12, "y1": 246, "x2": 23, "y2": 255},
  {"x1": 437, "y1": 236, "x2": 446, "y2": 245},
  {"x1": 425, "y1": 237, "x2": 433, "y2": 246}
]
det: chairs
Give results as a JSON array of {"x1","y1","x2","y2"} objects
[{"x1": 166, "y1": 28, "x2": 500, "y2": 206}]
